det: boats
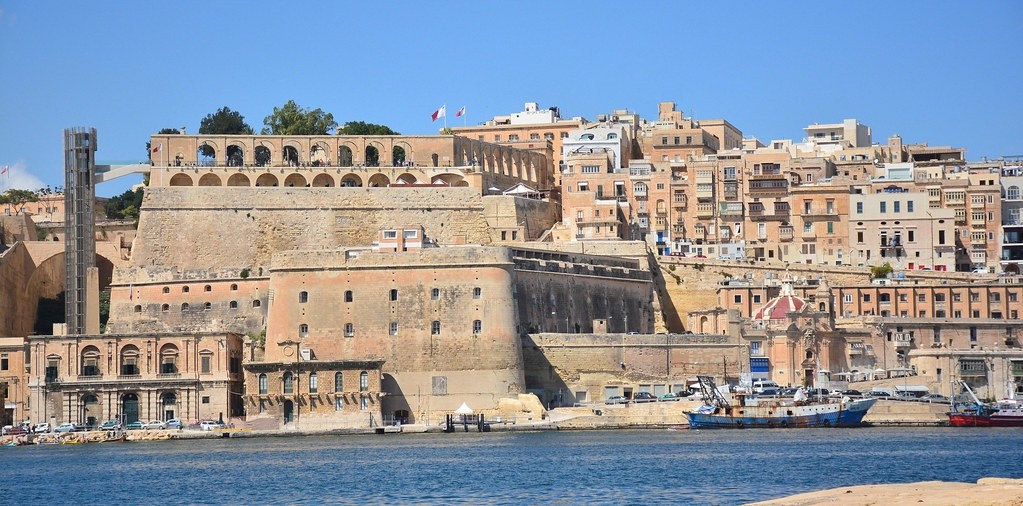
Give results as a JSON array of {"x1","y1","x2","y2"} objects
[
  {"x1": 943, "y1": 378, "x2": 1023, "y2": 426},
  {"x1": 681, "y1": 358, "x2": 879, "y2": 429}
]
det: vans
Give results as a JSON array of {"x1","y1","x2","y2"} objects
[
  {"x1": 754, "y1": 381, "x2": 781, "y2": 395},
  {"x1": 634, "y1": 392, "x2": 656, "y2": 402},
  {"x1": 972, "y1": 269, "x2": 989, "y2": 274}
]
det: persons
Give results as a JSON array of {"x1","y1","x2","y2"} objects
[{"x1": 27, "y1": 424, "x2": 38, "y2": 434}]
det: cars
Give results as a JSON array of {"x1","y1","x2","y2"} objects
[
  {"x1": 605, "y1": 395, "x2": 630, "y2": 404},
  {"x1": 200, "y1": 421, "x2": 220, "y2": 430},
  {"x1": 141, "y1": 420, "x2": 168, "y2": 429},
  {"x1": 774, "y1": 384, "x2": 968, "y2": 403},
  {"x1": 125, "y1": 422, "x2": 145, "y2": 430},
  {"x1": 165, "y1": 419, "x2": 183, "y2": 429},
  {"x1": 98, "y1": 420, "x2": 122, "y2": 430},
  {"x1": 188, "y1": 418, "x2": 226, "y2": 429},
  {"x1": 666, "y1": 251, "x2": 730, "y2": 260},
  {"x1": 687, "y1": 392, "x2": 709, "y2": 400},
  {"x1": 658, "y1": 392, "x2": 679, "y2": 401},
  {"x1": 678, "y1": 391, "x2": 692, "y2": 397},
  {"x1": 2, "y1": 421, "x2": 76, "y2": 435}
]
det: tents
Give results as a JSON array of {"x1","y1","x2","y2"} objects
[
  {"x1": 503, "y1": 183, "x2": 540, "y2": 199},
  {"x1": 454, "y1": 403, "x2": 473, "y2": 425}
]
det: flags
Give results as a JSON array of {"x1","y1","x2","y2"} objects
[
  {"x1": 431, "y1": 105, "x2": 445, "y2": 121},
  {"x1": 456, "y1": 106, "x2": 464, "y2": 117},
  {"x1": 153, "y1": 144, "x2": 161, "y2": 153},
  {"x1": 1, "y1": 165, "x2": 8, "y2": 174}
]
